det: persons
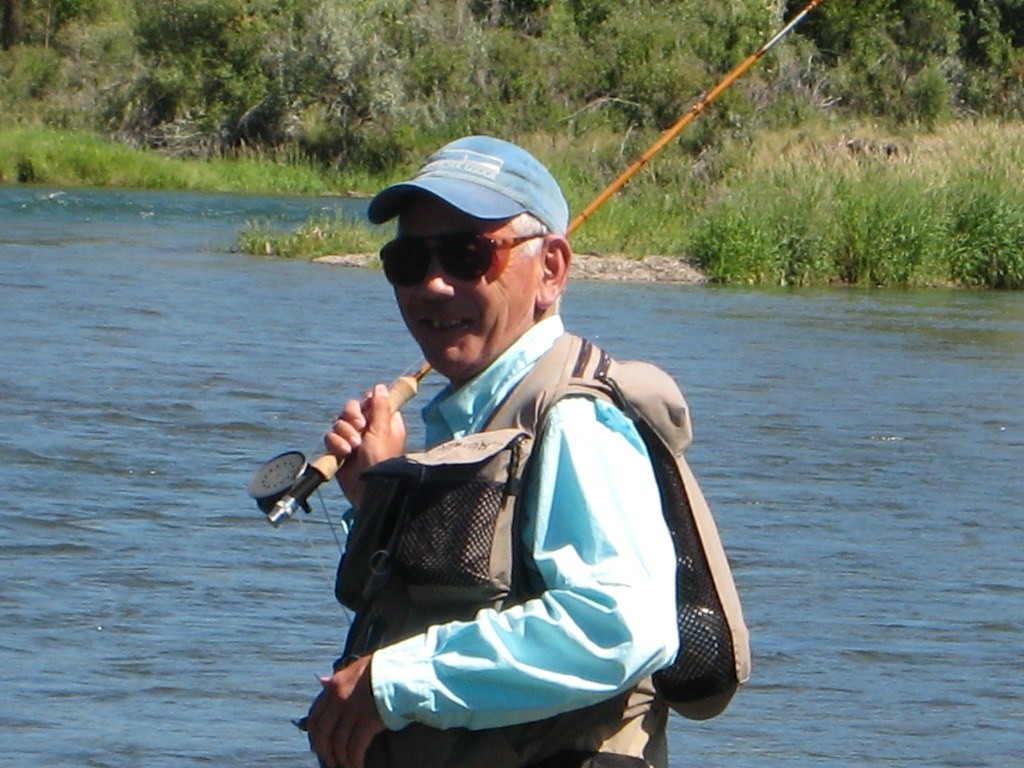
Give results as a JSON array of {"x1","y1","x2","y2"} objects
[{"x1": 289, "y1": 136, "x2": 753, "y2": 768}]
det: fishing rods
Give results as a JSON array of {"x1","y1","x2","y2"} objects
[{"x1": 247, "y1": 1, "x2": 818, "y2": 529}]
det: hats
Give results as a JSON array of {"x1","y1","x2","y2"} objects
[{"x1": 368, "y1": 135, "x2": 570, "y2": 234}]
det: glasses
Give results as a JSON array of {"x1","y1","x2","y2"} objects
[{"x1": 380, "y1": 234, "x2": 545, "y2": 289}]
